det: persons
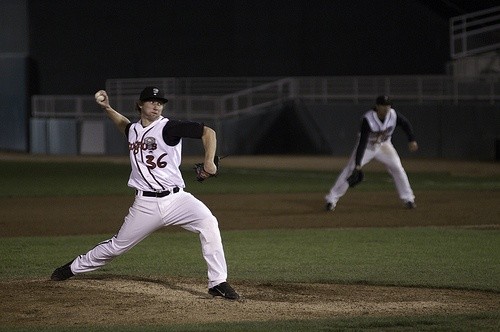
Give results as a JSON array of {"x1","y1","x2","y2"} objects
[
  {"x1": 325, "y1": 96, "x2": 417, "y2": 211},
  {"x1": 52, "y1": 87, "x2": 240, "y2": 300}
]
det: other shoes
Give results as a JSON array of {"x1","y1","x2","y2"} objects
[
  {"x1": 327, "y1": 203, "x2": 335, "y2": 212},
  {"x1": 407, "y1": 202, "x2": 416, "y2": 207}
]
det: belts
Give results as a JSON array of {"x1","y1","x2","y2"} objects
[{"x1": 135, "y1": 187, "x2": 179, "y2": 198}]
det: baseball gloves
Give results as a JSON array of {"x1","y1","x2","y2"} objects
[
  {"x1": 193, "y1": 155, "x2": 220, "y2": 182},
  {"x1": 346, "y1": 168, "x2": 363, "y2": 188}
]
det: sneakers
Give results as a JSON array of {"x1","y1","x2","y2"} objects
[
  {"x1": 51, "y1": 260, "x2": 75, "y2": 281},
  {"x1": 208, "y1": 282, "x2": 240, "y2": 300}
]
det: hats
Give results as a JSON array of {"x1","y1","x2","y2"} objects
[
  {"x1": 376, "y1": 96, "x2": 392, "y2": 105},
  {"x1": 139, "y1": 87, "x2": 168, "y2": 104}
]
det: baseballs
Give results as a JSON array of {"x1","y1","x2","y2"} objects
[{"x1": 97, "y1": 95, "x2": 105, "y2": 100}]
